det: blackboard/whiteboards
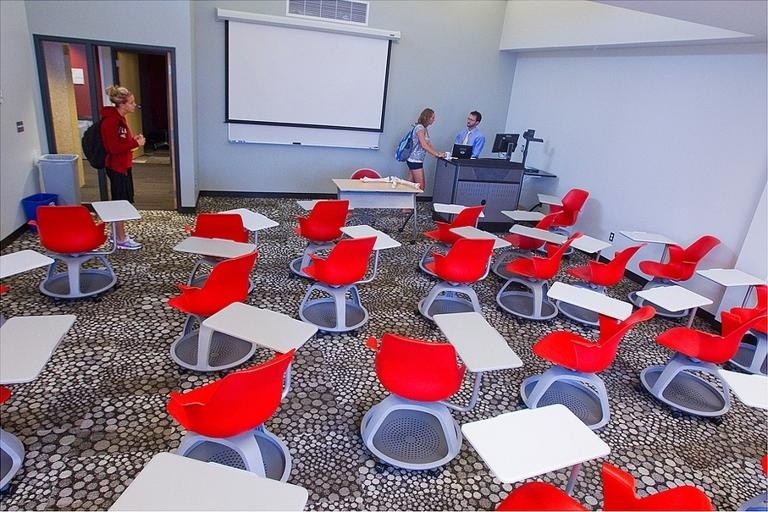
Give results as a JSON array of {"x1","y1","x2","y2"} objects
[{"x1": 228, "y1": 123, "x2": 380, "y2": 151}]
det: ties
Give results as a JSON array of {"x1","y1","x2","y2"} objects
[{"x1": 462, "y1": 131, "x2": 471, "y2": 144}]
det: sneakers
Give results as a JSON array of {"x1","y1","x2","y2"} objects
[{"x1": 110, "y1": 233, "x2": 143, "y2": 250}]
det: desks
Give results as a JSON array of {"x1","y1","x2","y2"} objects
[
  {"x1": 0, "y1": 249, "x2": 56, "y2": 279},
  {"x1": 448, "y1": 226, "x2": 512, "y2": 250},
  {"x1": 569, "y1": 231, "x2": 612, "y2": 261},
  {"x1": 501, "y1": 209, "x2": 548, "y2": 223},
  {"x1": 460, "y1": 402, "x2": 613, "y2": 492},
  {"x1": 339, "y1": 224, "x2": 403, "y2": 284},
  {"x1": 219, "y1": 208, "x2": 278, "y2": 248},
  {"x1": 636, "y1": 285, "x2": 713, "y2": 328},
  {"x1": 296, "y1": 198, "x2": 327, "y2": 212},
  {"x1": 1, "y1": 314, "x2": 78, "y2": 385},
  {"x1": 433, "y1": 309, "x2": 525, "y2": 411},
  {"x1": 431, "y1": 158, "x2": 524, "y2": 233},
  {"x1": 173, "y1": 236, "x2": 257, "y2": 260},
  {"x1": 695, "y1": 267, "x2": 767, "y2": 307},
  {"x1": 434, "y1": 203, "x2": 485, "y2": 227},
  {"x1": 509, "y1": 221, "x2": 567, "y2": 246},
  {"x1": 546, "y1": 281, "x2": 634, "y2": 323},
  {"x1": 332, "y1": 177, "x2": 424, "y2": 240},
  {"x1": 202, "y1": 301, "x2": 319, "y2": 399},
  {"x1": 108, "y1": 452, "x2": 310, "y2": 510},
  {"x1": 538, "y1": 193, "x2": 563, "y2": 205},
  {"x1": 620, "y1": 229, "x2": 684, "y2": 262},
  {"x1": 717, "y1": 367, "x2": 768, "y2": 410},
  {"x1": 524, "y1": 167, "x2": 555, "y2": 177},
  {"x1": 85, "y1": 197, "x2": 142, "y2": 255}
]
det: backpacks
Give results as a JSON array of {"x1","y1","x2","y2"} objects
[
  {"x1": 82, "y1": 117, "x2": 106, "y2": 169},
  {"x1": 395, "y1": 123, "x2": 419, "y2": 162}
]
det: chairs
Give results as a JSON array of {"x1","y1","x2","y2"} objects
[
  {"x1": 540, "y1": 188, "x2": 590, "y2": 257},
  {"x1": 628, "y1": 234, "x2": 720, "y2": 318},
  {"x1": 520, "y1": 305, "x2": 657, "y2": 431},
  {"x1": 494, "y1": 465, "x2": 713, "y2": 511},
  {"x1": 186, "y1": 213, "x2": 254, "y2": 294},
  {"x1": 727, "y1": 284, "x2": 768, "y2": 375},
  {"x1": 492, "y1": 211, "x2": 564, "y2": 284},
  {"x1": 419, "y1": 205, "x2": 486, "y2": 276},
  {"x1": 167, "y1": 349, "x2": 297, "y2": 483},
  {"x1": 298, "y1": 236, "x2": 378, "y2": 333},
  {"x1": 37, "y1": 207, "x2": 117, "y2": 301},
  {"x1": 417, "y1": 238, "x2": 496, "y2": 320},
  {"x1": 734, "y1": 455, "x2": 768, "y2": 511},
  {"x1": 347, "y1": 168, "x2": 389, "y2": 232},
  {"x1": 361, "y1": 336, "x2": 462, "y2": 471},
  {"x1": 0, "y1": 387, "x2": 25, "y2": 491},
  {"x1": 290, "y1": 199, "x2": 350, "y2": 279},
  {"x1": 640, "y1": 312, "x2": 746, "y2": 417},
  {"x1": 556, "y1": 244, "x2": 645, "y2": 326},
  {"x1": 167, "y1": 252, "x2": 256, "y2": 373}
]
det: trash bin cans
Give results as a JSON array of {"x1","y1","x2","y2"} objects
[
  {"x1": 37, "y1": 154, "x2": 80, "y2": 206},
  {"x1": 20, "y1": 193, "x2": 57, "y2": 233}
]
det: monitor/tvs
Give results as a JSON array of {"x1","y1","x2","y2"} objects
[
  {"x1": 452, "y1": 144, "x2": 473, "y2": 159},
  {"x1": 491, "y1": 133, "x2": 519, "y2": 160}
]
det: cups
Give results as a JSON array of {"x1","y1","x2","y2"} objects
[{"x1": 445, "y1": 152, "x2": 450, "y2": 159}]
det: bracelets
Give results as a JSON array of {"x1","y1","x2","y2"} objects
[{"x1": 432, "y1": 151, "x2": 436, "y2": 156}]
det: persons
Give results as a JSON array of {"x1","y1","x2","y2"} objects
[
  {"x1": 401, "y1": 108, "x2": 444, "y2": 214},
  {"x1": 449, "y1": 111, "x2": 485, "y2": 159},
  {"x1": 94, "y1": 85, "x2": 146, "y2": 251}
]
